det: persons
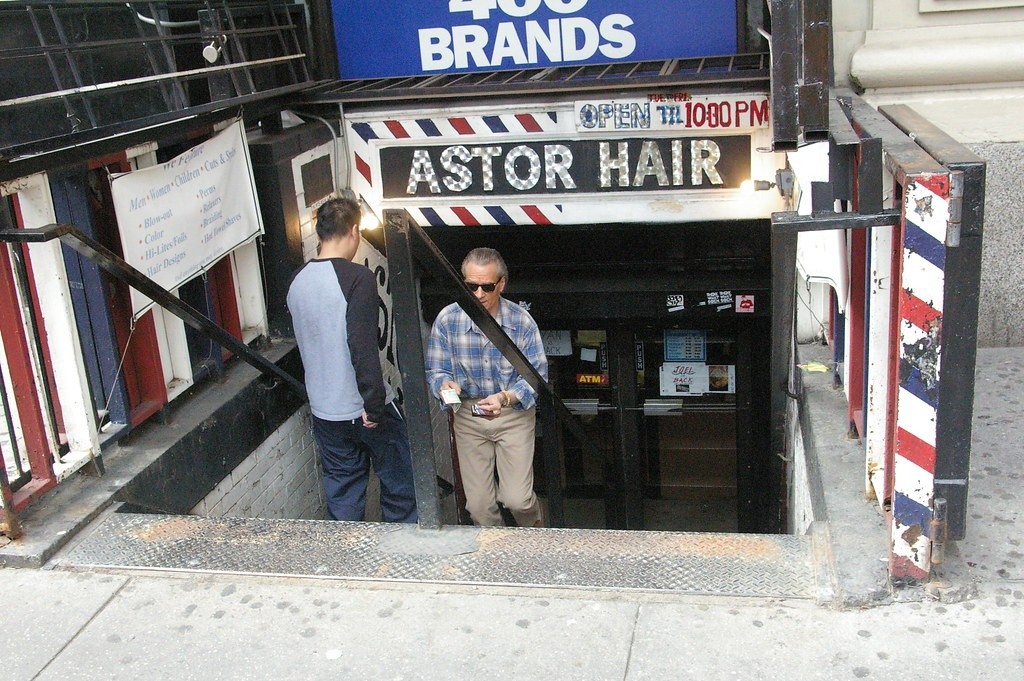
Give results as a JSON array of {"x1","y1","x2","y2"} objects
[
  {"x1": 424, "y1": 248, "x2": 548, "y2": 527},
  {"x1": 281, "y1": 198, "x2": 417, "y2": 523}
]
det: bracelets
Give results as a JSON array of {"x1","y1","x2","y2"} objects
[{"x1": 499, "y1": 389, "x2": 510, "y2": 408}]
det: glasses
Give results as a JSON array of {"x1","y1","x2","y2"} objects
[{"x1": 465, "y1": 276, "x2": 503, "y2": 292}]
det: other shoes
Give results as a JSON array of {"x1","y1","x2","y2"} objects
[{"x1": 530, "y1": 496, "x2": 545, "y2": 527}]
